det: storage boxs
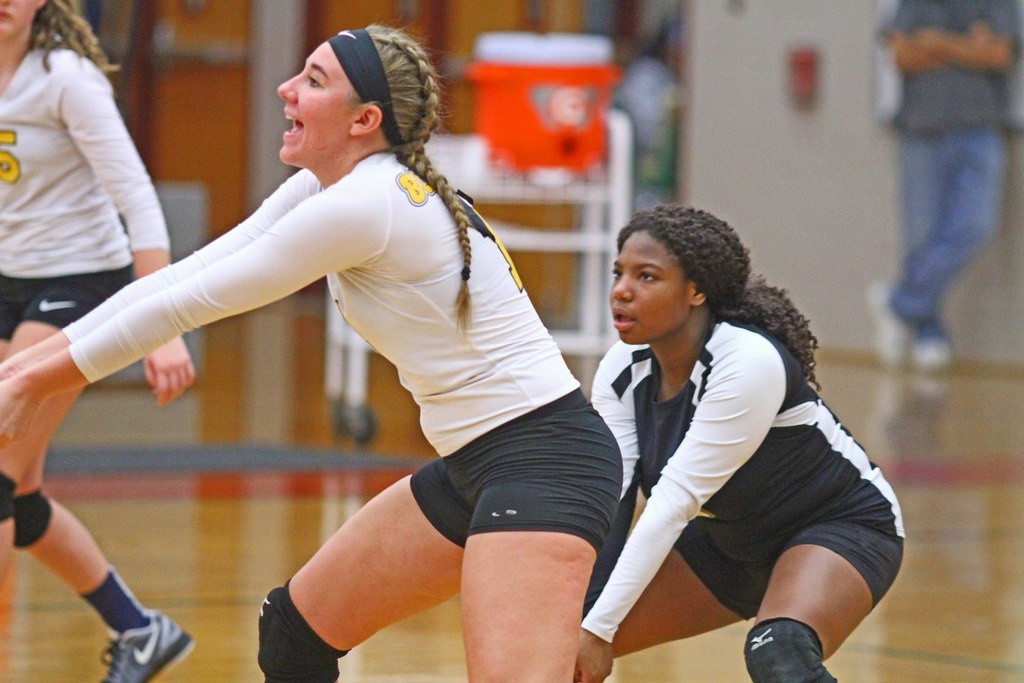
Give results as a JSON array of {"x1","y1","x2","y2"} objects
[{"x1": 461, "y1": 29, "x2": 625, "y2": 184}]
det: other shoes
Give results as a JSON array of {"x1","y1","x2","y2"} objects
[
  {"x1": 864, "y1": 280, "x2": 909, "y2": 366},
  {"x1": 911, "y1": 336, "x2": 954, "y2": 371}
]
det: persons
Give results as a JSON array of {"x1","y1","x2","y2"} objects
[
  {"x1": 589, "y1": 204, "x2": 905, "y2": 683},
  {"x1": 0, "y1": 0, "x2": 196, "y2": 683},
  {"x1": 866, "y1": 0, "x2": 1017, "y2": 370},
  {"x1": 0, "y1": 23, "x2": 624, "y2": 683}
]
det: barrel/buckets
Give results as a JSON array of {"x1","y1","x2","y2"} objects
[{"x1": 462, "y1": 30, "x2": 625, "y2": 178}]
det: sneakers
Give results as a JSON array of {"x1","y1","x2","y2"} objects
[{"x1": 96, "y1": 610, "x2": 197, "y2": 683}]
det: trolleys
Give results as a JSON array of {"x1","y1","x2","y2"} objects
[{"x1": 325, "y1": 103, "x2": 636, "y2": 448}]
